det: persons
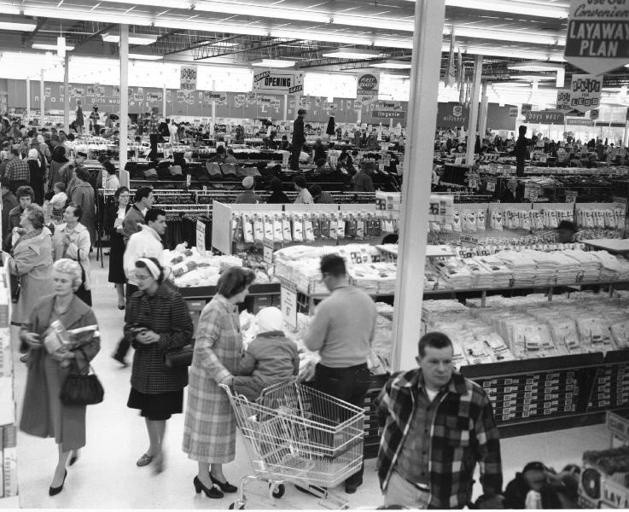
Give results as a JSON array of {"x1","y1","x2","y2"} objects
[
  {"x1": 308, "y1": 185, "x2": 333, "y2": 203},
  {"x1": 294, "y1": 177, "x2": 314, "y2": 204},
  {"x1": 124, "y1": 257, "x2": 194, "y2": 475},
  {"x1": 235, "y1": 177, "x2": 262, "y2": 203},
  {"x1": 266, "y1": 176, "x2": 290, "y2": 203},
  {"x1": 105, "y1": 187, "x2": 133, "y2": 310},
  {"x1": 52, "y1": 202, "x2": 93, "y2": 308},
  {"x1": 50, "y1": 181, "x2": 67, "y2": 220},
  {"x1": 123, "y1": 187, "x2": 154, "y2": 247},
  {"x1": 18, "y1": 258, "x2": 102, "y2": 496},
  {"x1": 305, "y1": 255, "x2": 379, "y2": 493},
  {"x1": 375, "y1": 332, "x2": 503, "y2": 509},
  {"x1": 514, "y1": 180, "x2": 526, "y2": 203},
  {"x1": 312, "y1": 125, "x2": 408, "y2": 187},
  {"x1": 183, "y1": 266, "x2": 256, "y2": 499},
  {"x1": 234, "y1": 305, "x2": 299, "y2": 422},
  {"x1": 0, "y1": 203, "x2": 55, "y2": 361},
  {"x1": 256, "y1": 119, "x2": 289, "y2": 151},
  {"x1": 208, "y1": 146, "x2": 225, "y2": 164},
  {"x1": 225, "y1": 148, "x2": 238, "y2": 164},
  {"x1": 163, "y1": 116, "x2": 246, "y2": 146},
  {"x1": 145, "y1": 107, "x2": 159, "y2": 161},
  {"x1": 9, "y1": 185, "x2": 34, "y2": 233},
  {"x1": 111, "y1": 209, "x2": 167, "y2": 369},
  {"x1": 513, "y1": 126, "x2": 539, "y2": 176},
  {"x1": 289, "y1": 109, "x2": 307, "y2": 170},
  {"x1": 1, "y1": 107, "x2": 121, "y2": 181},
  {"x1": 431, "y1": 126, "x2": 513, "y2": 203},
  {"x1": 542, "y1": 135, "x2": 629, "y2": 168},
  {"x1": 70, "y1": 168, "x2": 95, "y2": 253}
]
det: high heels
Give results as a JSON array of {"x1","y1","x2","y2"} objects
[
  {"x1": 193, "y1": 475, "x2": 225, "y2": 500},
  {"x1": 208, "y1": 469, "x2": 240, "y2": 495},
  {"x1": 67, "y1": 451, "x2": 79, "y2": 467},
  {"x1": 48, "y1": 468, "x2": 68, "y2": 497}
]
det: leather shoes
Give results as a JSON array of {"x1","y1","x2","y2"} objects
[
  {"x1": 135, "y1": 451, "x2": 153, "y2": 467},
  {"x1": 110, "y1": 352, "x2": 129, "y2": 368},
  {"x1": 117, "y1": 302, "x2": 125, "y2": 312},
  {"x1": 342, "y1": 475, "x2": 363, "y2": 495},
  {"x1": 151, "y1": 453, "x2": 164, "y2": 472}
]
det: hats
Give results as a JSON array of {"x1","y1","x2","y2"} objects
[{"x1": 241, "y1": 175, "x2": 254, "y2": 189}]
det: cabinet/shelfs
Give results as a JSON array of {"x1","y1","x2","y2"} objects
[
  {"x1": 169, "y1": 198, "x2": 629, "y2": 301},
  {"x1": 271, "y1": 242, "x2": 629, "y2": 459}
]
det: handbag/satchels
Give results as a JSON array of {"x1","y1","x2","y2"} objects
[
  {"x1": 161, "y1": 335, "x2": 195, "y2": 370},
  {"x1": 9, "y1": 263, "x2": 23, "y2": 305},
  {"x1": 58, "y1": 347, "x2": 106, "y2": 406}
]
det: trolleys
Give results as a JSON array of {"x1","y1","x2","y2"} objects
[{"x1": 216, "y1": 383, "x2": 365, "y2": 510}]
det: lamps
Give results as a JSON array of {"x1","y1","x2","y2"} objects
[
  {"x1": 0, "y1": 13, "x2": 43, "y2": 34},
  {"x1": 322, "y1": 46, "x2": 390, "y2": 62},
  {"x1": 508, "y1": 60, "x2": 568, "y2": 73},
  {"x1": 99, "y1": 27, "x2": 164, "y2": 48},
  {"x1": 251, "y1": 57, "x2": 298, "y2": 71},
  {"x1": 126, "y1": 49, "x2": 166, "y2": 63},
  {"x1": 368, "y1": 60, "x2": 411, "y2": 71}
]
